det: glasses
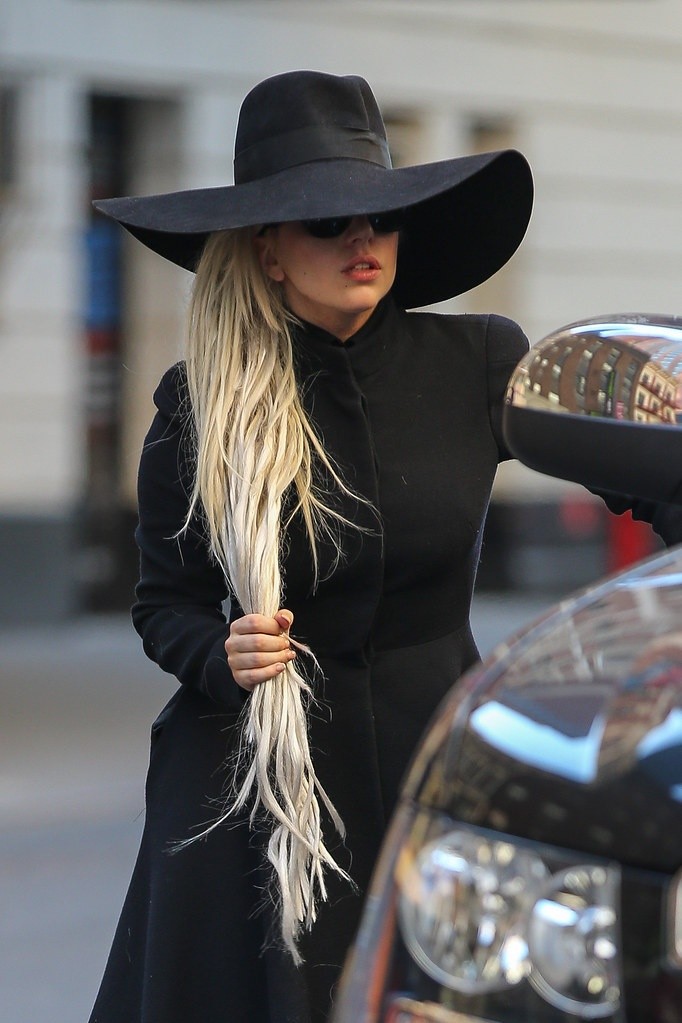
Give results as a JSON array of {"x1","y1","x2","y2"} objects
[{"x1": 254, "y1": 211, "x2": 409, "y2": 241}]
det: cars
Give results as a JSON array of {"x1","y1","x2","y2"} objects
[{"x1": 325, "y1": 312, "x2": 682, "y2": 1023}]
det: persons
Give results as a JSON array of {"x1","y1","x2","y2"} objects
[{"x1": 87, "y1": 70, "x2": 681, "y2": 1023}]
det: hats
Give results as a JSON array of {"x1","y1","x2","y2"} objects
[{"x1": 94, "y1": 67, "x2": 536, "y2": 316}]
